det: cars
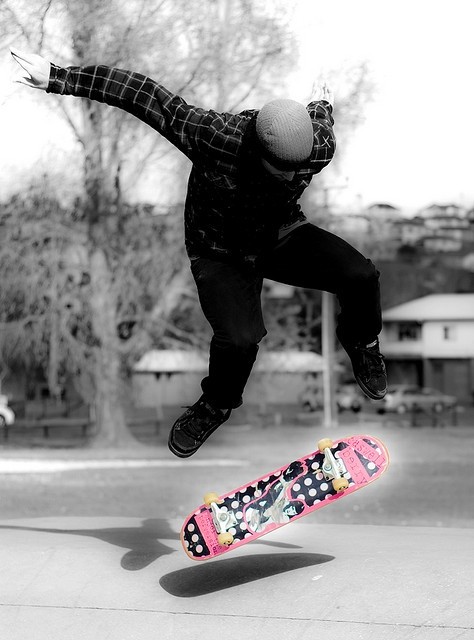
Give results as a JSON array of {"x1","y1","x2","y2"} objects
[
  {"x1": 0, "y1": 394, "x2": 16, "y2": 436},
  {"x1": 300, "y1": 385, "x2": 363, "y2": 413},
  {"x1": 370, "y1": 384, "x2": 456, "y2": 415}
]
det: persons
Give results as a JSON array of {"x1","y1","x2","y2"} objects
[{"x1": 9, "y1": 47, "x2": 387, "y2": 459}]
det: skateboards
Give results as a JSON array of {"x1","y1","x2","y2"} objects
[{"x1": 179, "y1": 434, "x2": 389, "y2": 561}]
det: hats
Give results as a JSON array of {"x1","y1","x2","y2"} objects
[{"x1": 255, "y1": 98, "x2": 314, "y2": 162}]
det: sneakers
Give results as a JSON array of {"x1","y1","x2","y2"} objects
[
  {"x1": 168, "y1": 393, "x2": 232, "y2": 458},
  {"x1": 336, "y1": 323, "x2": 388, "y2": 401}
]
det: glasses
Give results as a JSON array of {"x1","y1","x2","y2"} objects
[{"x1": 256, "y1": 133, "x2": 314, "y2": 172}]
